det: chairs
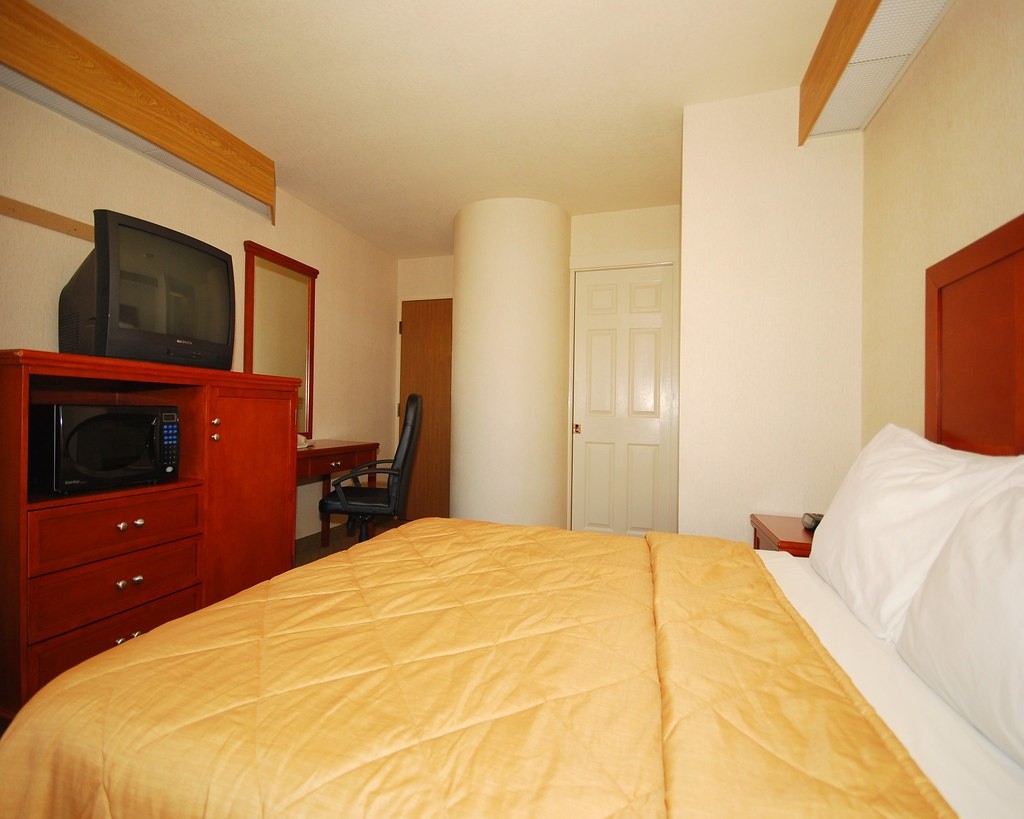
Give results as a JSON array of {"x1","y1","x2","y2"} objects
[{"x1": 319, "y1": 393, "x2": 423, "y2": 543}]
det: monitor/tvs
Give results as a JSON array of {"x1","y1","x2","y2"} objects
[{"x1": 58, "y1": 208, "x2": 235, "y2": 371}]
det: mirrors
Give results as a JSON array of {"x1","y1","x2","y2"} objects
[{"x1": 243, "y1": 240, "x2": 321, "y2": 440}]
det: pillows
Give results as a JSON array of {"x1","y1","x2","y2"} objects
[{"x1": 810, "y1": 423, "x2": 1024, "y2": 765}]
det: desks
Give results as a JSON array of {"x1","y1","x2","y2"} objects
[{"x1": 294, "y1": 440, "x2": 380, "y2": 548}]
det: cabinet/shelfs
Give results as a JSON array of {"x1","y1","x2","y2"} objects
[{"x1": 0, "y1": 349, "x2": 304, "y2": 736}]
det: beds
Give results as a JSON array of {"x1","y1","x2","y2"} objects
[{"x1": 0, "y1": 212, "x2": 1024, "y2": 819}]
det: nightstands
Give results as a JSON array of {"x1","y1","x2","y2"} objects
[{"x1": 750, "y1": 514, "x2": 816, "y2": 558}]
